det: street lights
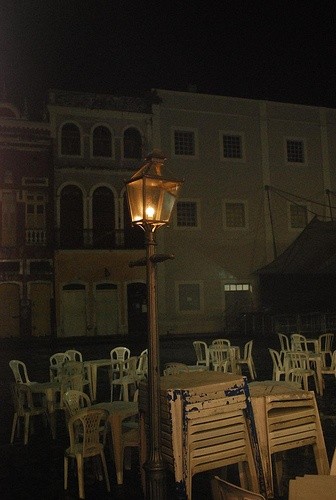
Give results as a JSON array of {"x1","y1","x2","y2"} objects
[{"x1": 120, "y1": 149, "x2": 185, "y2": 500}]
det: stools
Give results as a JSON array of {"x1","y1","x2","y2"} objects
[{"x1": 157, "y1": 371, "x2": 330, "y2": 500}]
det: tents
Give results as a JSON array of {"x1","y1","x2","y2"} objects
[{"x1": 259, "y1": 216, "x2": 335, "y2": 311}]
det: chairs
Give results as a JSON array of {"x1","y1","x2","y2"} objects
[
  {"x1": 64, "y1": 351, "x2": 82, "y2": 363},
  {"x1": 50, "y1": 354, "x2": 69, "y2": 383},
  {"x1": 10, "y1": 361, "x2": 31, "y2": 384},
  {"x1": 58, "y1": 360, "x2": 84, "y2": 412},
  {"x1": 208, "y1": 344, "x2": 235, "y2": 375},
  {"x1": 319, "y1": 333, "x2": 334, "y2": 367},
  {"x1": 268, "y1": 348, "x2": 285, "y2": 383},
  {"x1": 62, "y1": 412, "x2": 111, "y2": 500},
  {"x1": 279, "y1": 332, "x2": 291, "y2": 368},
  {"x1": 290, "y1": 334, "x2": 308, "y2": 354},
  {"x1": 212, "y1": 339, "x2": 230, "y2": 347},
  {"x1": 110, "y1": 356, "x2": 137, "y2": 403},
  {"x1": 110, "y1": 349, "x2": 131, "y2": 388},
  {"x1": 193, "y1": 340, "x2": 210, "y2": 371},
  {"x1": 235, "y1": 340, "x2": 257, "y2": 379},
  {"x1": 285, "y1": 353, "x2": 319, "y2": 393},
  {"x1": 66, "y1": 391, "x2": 107, "y2": 449},
  {"x1": 10, "y1": 383, "x2": 48, "y2": 447},
  {"x1": 321, "y1": 351, "x2": 336, "y2": 383},
  {"x1": 137, "y1": 349, "x2": 150, "y2": 379}
]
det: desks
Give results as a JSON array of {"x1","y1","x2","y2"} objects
[
  {"x1": 299, "y1": 338, "x2": 318, "y2": 353},
  {"x1": 209, "y1": 345, "x2": 242, "y2": 375},
  {"x1": 285, "y1": 351, "x2": 325, "y2": 388},
  {"x1": 82, "y1": 359, "x2": 128, "y2": 402}
]
